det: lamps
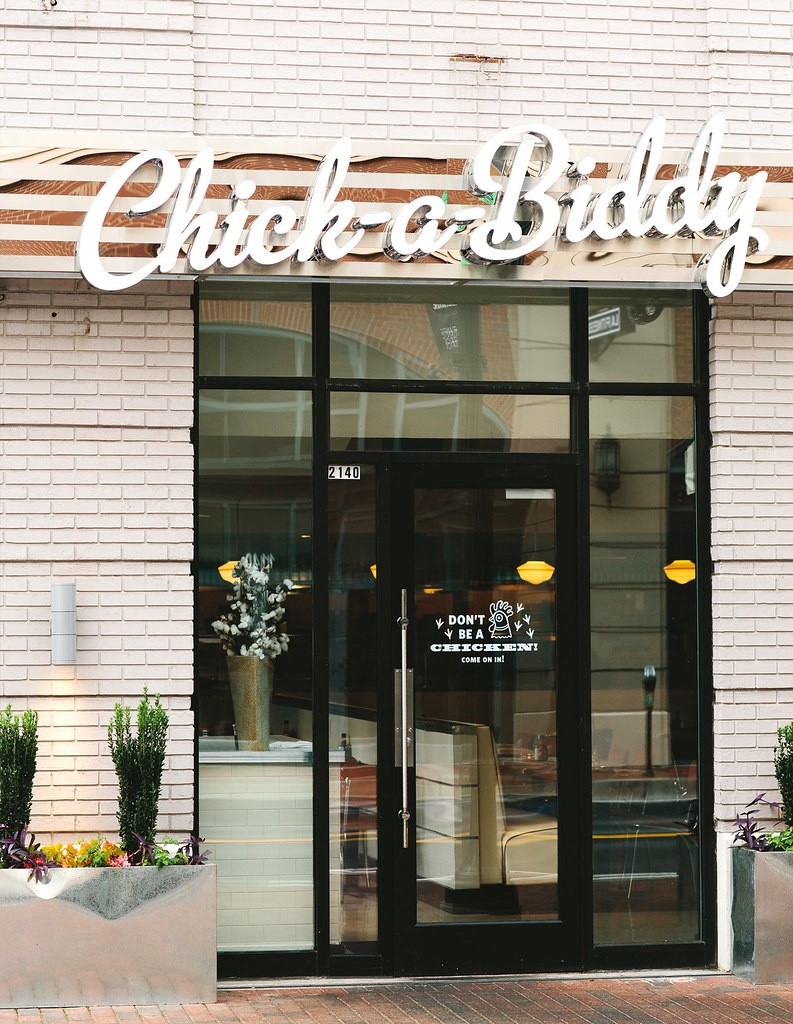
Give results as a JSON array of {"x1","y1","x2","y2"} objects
[
  {"x1": 663, "y1": 559, "x2": 696, "y2": 585},
  {"x1": 592, "y1": 420, "x2": 622, "y2": 513},
  {"x1": 517, "y1": 500, "x2": 556, "y2": 586},
  {"x1": 51, "y1": 582, "x2": 76, "y2": 665}
]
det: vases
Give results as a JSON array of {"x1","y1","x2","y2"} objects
[{"x1": 227, "y1": 657, "x2": 275, "y2": 747}]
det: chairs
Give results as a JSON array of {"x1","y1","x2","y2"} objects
[{"x1": 341, "y1": 764, "x2": 375, "y2": 903}]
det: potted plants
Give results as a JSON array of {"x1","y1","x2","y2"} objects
[
  {"x1": 729, "y1": 719, "x2": 793, "y2": 985},
  {"x1": 1, "y1": 681, "x2": 219, "y2": 1007}
]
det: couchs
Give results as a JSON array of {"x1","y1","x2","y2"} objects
[
  {"x1": 591, "y1": 778, "x2": 697, "y2": 900},
  {"x1": 275, "y1": 692, "x2": 558, "y2": 913}
]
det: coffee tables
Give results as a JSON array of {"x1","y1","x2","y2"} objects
[{"x1": 506, "y1": 758, "x2": 697, "y2": 904}]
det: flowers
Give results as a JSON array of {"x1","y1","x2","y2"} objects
[{"x1": 211, "y1": 549, "x2": 292, "y2": 657}]
